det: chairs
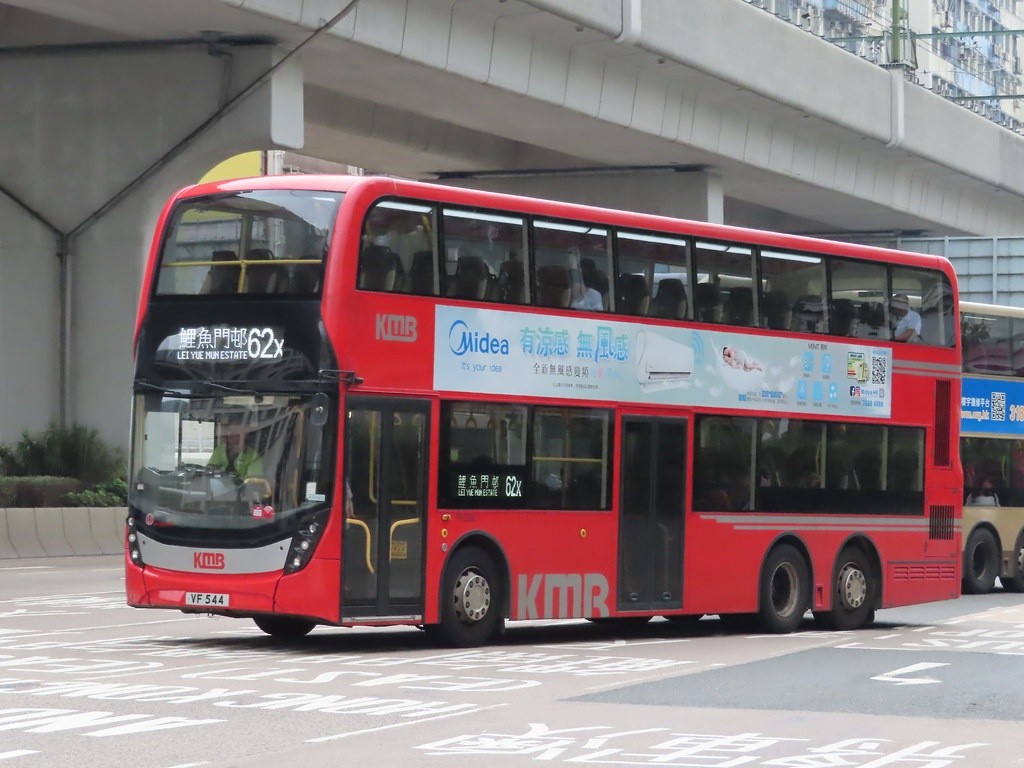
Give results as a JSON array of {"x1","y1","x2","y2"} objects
[{"x1": 196, "y1": 249, "x2": 893, "y2": 341}]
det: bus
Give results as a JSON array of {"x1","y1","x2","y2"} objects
[
  {"x1": 624, "y1": 274, "x2": 1023, "y2": 595},
  {"x1": 124, "y1": 170, "x2": 963, "y2": 650}
]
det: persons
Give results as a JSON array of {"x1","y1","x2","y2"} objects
[
  {"x1": 722, "y1": 356, "x2": 740, "y2": 368},
  {"x1": 965, "y1": 478, "x2": 1001, "y2": 508},
  {"x1": 723, "y1": 347, "x2": 762, "y2": 372},
  {"x1": 206, "y1": 269, "x2": 924, "y2": 489}
]
